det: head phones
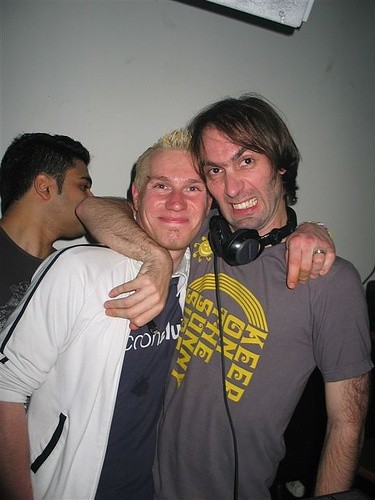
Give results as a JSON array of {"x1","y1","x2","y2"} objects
[{"x1": 208, "y1": 205, "x2": 297, "y2": 266}]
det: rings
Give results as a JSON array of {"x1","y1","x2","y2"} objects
[{"x1": 313, "y1": 250, "x2": 325, "y2": 254}]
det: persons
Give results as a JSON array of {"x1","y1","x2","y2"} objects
[
  {"x1": 73, "y1": 92, "x2": 374, "y2": 500},
  {"x1": 0, "y1": 132, "x2": 95, "y2": 336},
  {"x1": 0, "y1": 128, "x2": 212, "y2": 500}
]
